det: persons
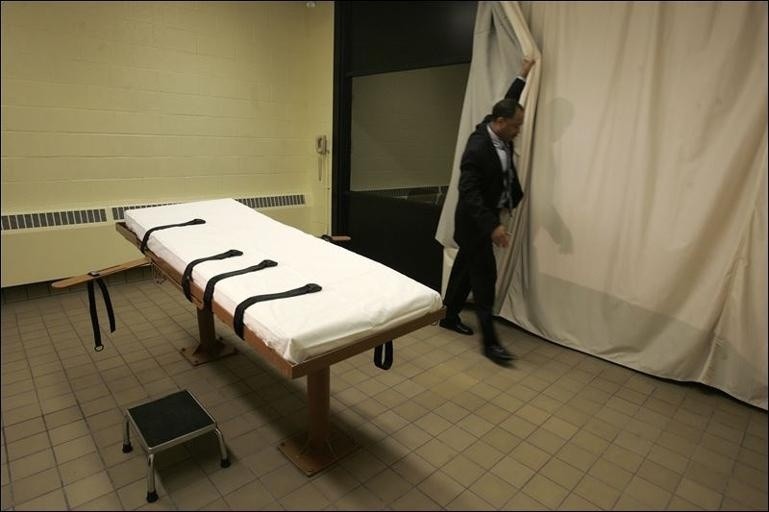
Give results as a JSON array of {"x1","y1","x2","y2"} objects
[{"x1": 440, "y1": 57, "x2": 537, "y2": 360}]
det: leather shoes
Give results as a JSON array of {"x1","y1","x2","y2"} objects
[
  {"x1": 439, "y1": 316, "x2": 473, "y2": 335},
  {"x1": 485, "y1": 343, "x2": 517, "y2": 362}
]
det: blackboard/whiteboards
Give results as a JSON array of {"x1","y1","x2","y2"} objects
[{"x1": 350, "y1": 65, "x2": 468, "y2": 192}]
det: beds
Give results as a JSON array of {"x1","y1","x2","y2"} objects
[{"x1": 112, "y1": 197, "x2": 450, "y2": 477}]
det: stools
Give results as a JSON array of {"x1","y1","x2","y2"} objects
[{"x1": 120, "y1": 390, "x2": 232, "y2": 503}]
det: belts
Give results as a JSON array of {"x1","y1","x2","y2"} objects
[{"x1": 87, "y1": 271, "x2": 115, "y2": 351}]
